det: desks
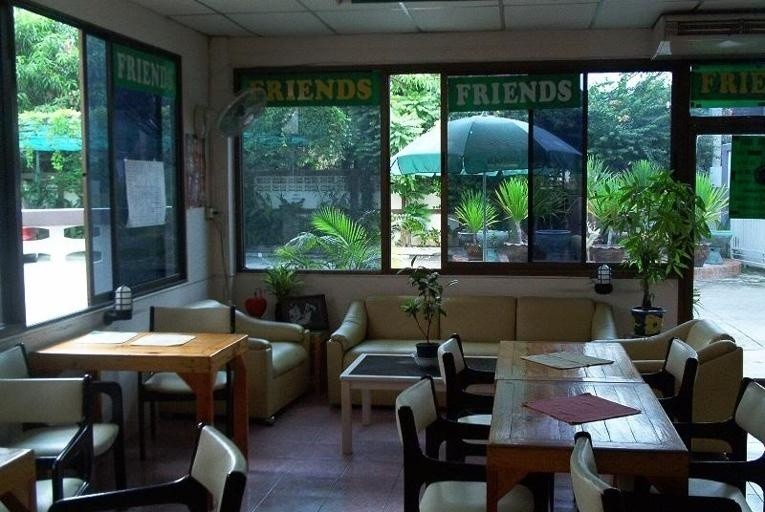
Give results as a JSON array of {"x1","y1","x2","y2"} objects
[
  {"x1": 486, "y1": 380, "x2": 694, "y2": 511},
  {"x1": 30, "y1": 326, "x2": 252, "y2": 477},
  {"x1": 0, "y1": 445, "x2": 40, "y2": 511},
  {"x1": 492, "y1": 336, "x2": 644, "y2": 382},
  {"x1": 306, "y1": 326, "x2": 329, "y2": 395}
]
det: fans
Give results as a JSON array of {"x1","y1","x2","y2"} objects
[{"x1": 192, "y1": 85, "x2": 268, "y2": 146}]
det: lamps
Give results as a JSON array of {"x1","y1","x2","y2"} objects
[
  {"x1": 588, "y1": 264, "x2": 615, "y2": 295},
  {"x1": 101, "y1": 284, "x2": 137, "y2": 322}
]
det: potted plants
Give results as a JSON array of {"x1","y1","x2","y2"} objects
[
  {"x1": 579, "y1": 179, "x2": 628, "y2": 266},
  {"x1": 444, "y1": 184, "x2": 499, "y2": 261},
  {"x1": 689, "y1": 167, "x2": 732, "y2": 271},
  {"x1": 491, "y1": 173, "x2": 532, "y2": 263},
  {"x1": 608, "y1": 159, "x2": 710, "y2": 338},
  {"x1": 392, "y1": 252, "x2": 451, "y2": 360}
]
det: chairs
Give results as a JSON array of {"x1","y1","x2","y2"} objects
[
  {"x1": 634, "y1": 332, "x2": 700, "y2": 453},
  {"x1": 179, "y1": 297, "x2": 313, "y2": 429},
  {"x1": 1, "y1": 342, "x2": 129, "y2": 489},
  {"x1": 433, "y1": 330, "x2": 497, "y2": 464},
  {"x1": 393, "y1": 373, "x2": 557, "y2": 512},
  {"x1": 568, "y1": 429, "x2": 744, "y2": 512},
  {"x1": 667, "y1": 374, "x2": 765, "y2": 511},
  {"x1": 1, "y1": 373, "x2": 98, "y2": 511},
  {"x1": 587, "y1": 315, "x2": 746, "y2": 460},
  {"x1": 136, "y1": 305, "x2": 240, "y2": 462},
  {"x1": 47, "y1": 420, "x2": 249, "y2": 512}
]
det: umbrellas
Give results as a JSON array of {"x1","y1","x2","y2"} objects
[{"x1": 389, "y1": 111, "x2": 582, "y2": 262}]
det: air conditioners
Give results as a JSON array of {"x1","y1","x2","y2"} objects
[{"x1": 645, "y1": 7, "x2": 765, "y2": 61}]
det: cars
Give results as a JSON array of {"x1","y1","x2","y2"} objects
[{"x1": 21, "y1": 196, "x2": 41, "y2": 262}]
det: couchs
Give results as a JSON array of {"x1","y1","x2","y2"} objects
[{"x1": 318, "y1": 291, "x2": 618, "y2": 413}]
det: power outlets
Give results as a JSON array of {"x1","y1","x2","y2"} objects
[{"x1": 206, "y1": 207, "x2": 219, "y2": 219}]
